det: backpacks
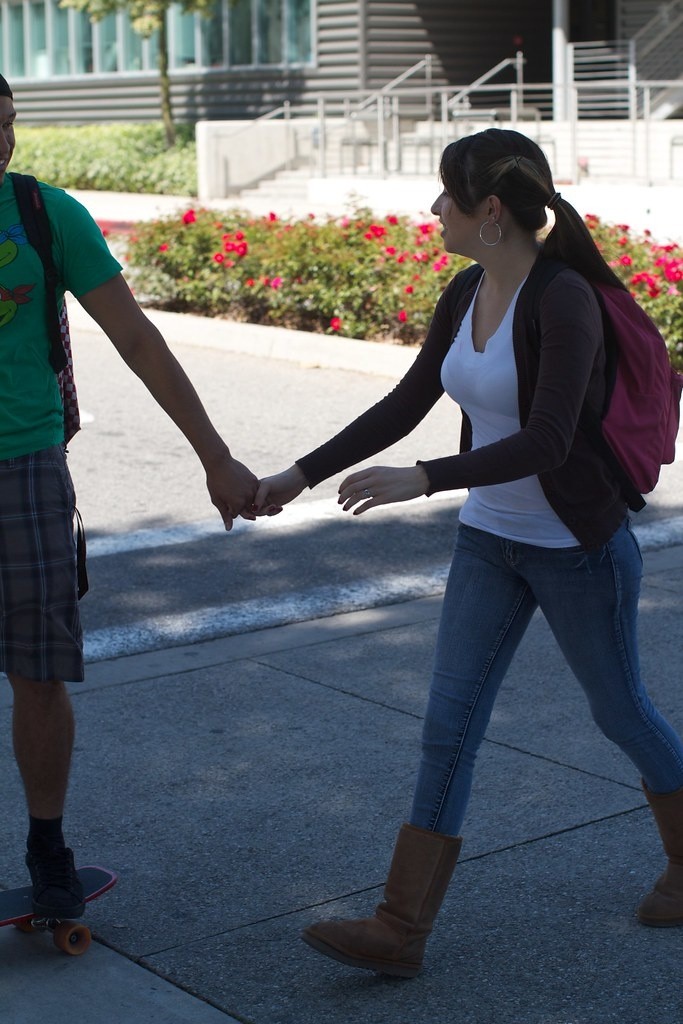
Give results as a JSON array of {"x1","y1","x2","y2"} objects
[
  {"x1": 9, "y1": 172, "x2": 82, "y2": 449},
  {"x1": 449, "y1": 264, "x2": 683, "y2": 512}
]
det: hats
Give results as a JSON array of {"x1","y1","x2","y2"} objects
[{"x1": 0, "y1": 73, "x2": 13, "y2": 100}]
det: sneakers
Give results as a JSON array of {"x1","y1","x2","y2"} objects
[{"x1": 25, "y1": 847, "x2": 86, "y2": 918}]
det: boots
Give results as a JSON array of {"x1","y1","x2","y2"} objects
[
  {"x1": 637, "y1": 776, "x2": 683, "y2": 926},
  {"x1": 302, "y1": 824, "x2": 462, "y2": 978}
]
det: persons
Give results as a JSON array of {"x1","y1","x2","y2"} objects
[
  {"x1": 0, "y1": 76, "x2": 261, "y2": 914},
  {"x1": 246, "y1": 126, "x2": 683, "y2": 976}
]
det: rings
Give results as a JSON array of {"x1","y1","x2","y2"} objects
[{"x1": 363, "y1": 489, "x2": 371, "y2": 500}]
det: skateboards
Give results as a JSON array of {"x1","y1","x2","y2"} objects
[{"x1": 1, "y1": 864, "x2": 119, "y2": 957}]
